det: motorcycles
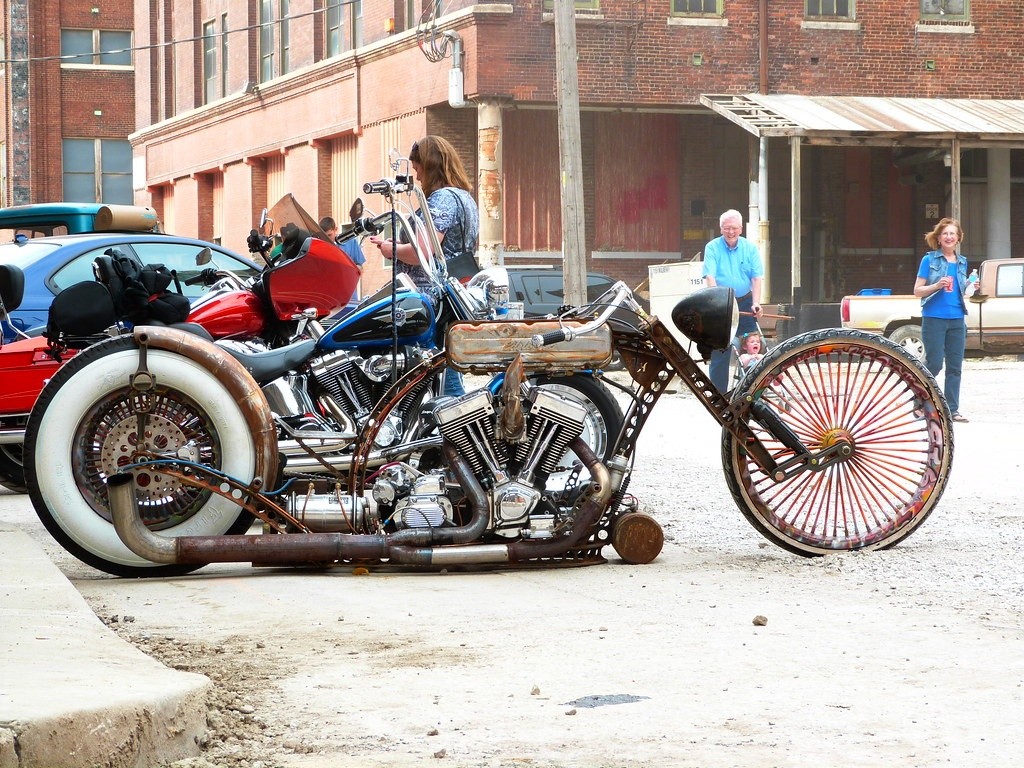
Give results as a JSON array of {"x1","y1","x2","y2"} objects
[{"x1": 1, "y1": 217, "x2": 954, "y2": 580}]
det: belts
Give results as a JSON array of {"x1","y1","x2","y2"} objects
[{"x1": 737, "y1": 291, "x2": 752, "y2": 302}]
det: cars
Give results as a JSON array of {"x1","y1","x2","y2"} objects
[{"x1": 2, "y1": 231, "x2": 264, "y2": 342}]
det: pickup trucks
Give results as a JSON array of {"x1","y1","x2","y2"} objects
[{"x1": 839, "y1": 259, "x2": 1024, "y2": 366}]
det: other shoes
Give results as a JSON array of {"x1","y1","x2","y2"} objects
[{"x1": 773, "y1": 374, "x2": 783, "y2": 386}]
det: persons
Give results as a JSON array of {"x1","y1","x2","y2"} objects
[
  {"x1": 318, "y1": 217, "x2": 367, "y2": 302},
  {"x1": 269, "y1": 222, "x2": 299, "y2": 263},
  {"x1": 702, "y1": 209, "x2": 764, "y2": 396},
  {"x1": 369, "y1": 134, "x2": 479, "y2": 398},
  {"x1": 739, "y1": 333, "x2": 784, "y2": 386},
  {"x1": 914, "y1": 218, "x2": 981, "y2": 423}
]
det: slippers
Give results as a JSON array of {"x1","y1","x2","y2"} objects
[
  {"x1": 951, "y1": 412, "x2": 969, "y2": 423},
  {"x1": 913, "y1": 400, "x2": 924, "y2": 418}
]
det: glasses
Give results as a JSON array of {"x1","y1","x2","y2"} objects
[{"x1": 412, "y1": 142, "x2": 418, "y2": 151}]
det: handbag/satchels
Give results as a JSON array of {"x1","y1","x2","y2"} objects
[
  {"x1": 442, "y1": 189, "x2": 481, "y2": 287},
  {"x1": 42, "y1": 281, "x2": 118, "y2": 363}
]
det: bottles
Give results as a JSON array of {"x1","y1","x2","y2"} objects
[{"x1": 969, "y1": 269, "x2": 978, "y2": 284}]
